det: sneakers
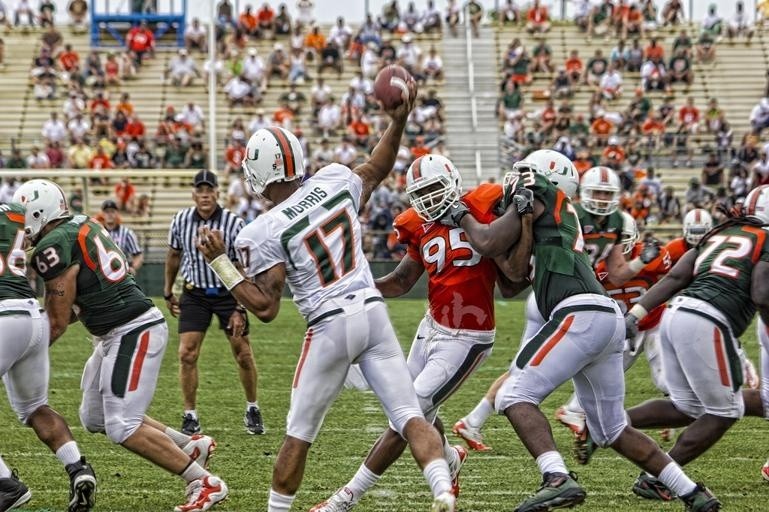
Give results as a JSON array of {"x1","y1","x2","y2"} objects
[
  {"x1": 181, "y1": 435, "x2": 218, "y2": 470},
  {"x1": 0, "y1": 468, "x2": 32, "y2": 511},
  {"x1": 174, "y1": 475, "x2": 228, "y2": 512},
  {"x1": 181, "y1": 413, "x2": 202, "y2": 437},
  {"x1": 243, "y1": 406, "x2": 266, "y2": 435},
  {"x1": 65, "y1": 455, "x2": 97, "y2": 512}
]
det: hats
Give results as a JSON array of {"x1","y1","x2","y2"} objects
[
  {"x1": 192, "y1": 169, "x2": 217, "y2": 188},
  {"x1": 102, "y1": 199, "x2": 117, "y2": 210}
]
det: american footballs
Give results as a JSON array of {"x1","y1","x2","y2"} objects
[{"x1": 374, "y1": 64, "x2": 414, "y2": 108}]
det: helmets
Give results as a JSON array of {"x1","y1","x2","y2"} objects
[
  {"x1": 683, "y1": 208, "x2": 713, "y2": 246},
  {"x1": 740, "y1": 184, "x2": 769, "y2": 225},
  {"x1": 622, "y1": 212, "x2": 640, "y2": 256},
  {"x1": 11, "y1": 178, "x2": 69, "y2": 248},
  {"x1": 241, "y1": 128, "x2": 304, "y2": 205},
  {"x1": 404, "y1": 154, "x2": 461, "y2": 223},
  {"x1": 580, "y1": 167, "x2": 621, "y2": 217},
  {"x1": 503, "y1": 148, "x2": 579, "y2": 202}
]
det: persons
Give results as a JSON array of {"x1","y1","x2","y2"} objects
[
  {"x1": 11, "y1": 178, "x2": 229, "y2": 512},
  {"x1": 576, "y1": 184, "x2": 769, "y2": 481},
  {"x1": 1, "y1": 0, "x2": 286, "y2": 278},
  {"x1": 309, "y1": 153, "x2": 536, "y2": 512},
  {"x1": 582, "y1": 0, "x2": 769, "y2": 230},
  {"x1": 494, "y1": 147, "x2": 724, "y2": 511},
  {"x1": 164, "y1": 170, "x2": 267, "y2": 436},
  {"x1": 191, "y1": 61, "x2": 456, "y2": 511},
  {"x1": 285, "y1": 0, "x2": 584, "y2": 261},
  {"x1": 452, "y1": 164, "x2": 662, "y2": 452},
  {"x1": 0, "y1": 167, "x2": 100, "y2": 512},
  {"x1": 571, "y1": 183, "x2": 769, "y2": 501}
]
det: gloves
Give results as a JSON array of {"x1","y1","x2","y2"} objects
[
  {"x1": 435, "y1": 201, "x2": 470, "y2": 228},
  {"x1": 512, "y1": 187, "x2": 535, "y2": 216}
]
